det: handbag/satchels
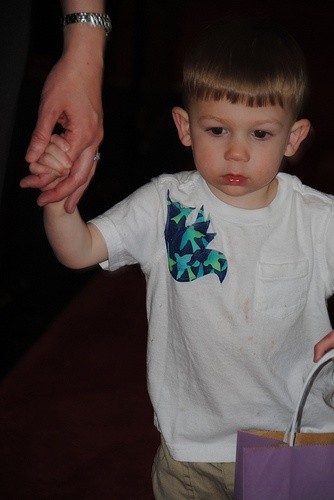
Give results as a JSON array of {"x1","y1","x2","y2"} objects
[{"x1": 233, "y1": 350, "x2": 334, "y2": 500}]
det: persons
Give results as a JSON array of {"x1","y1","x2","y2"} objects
[
  {"x1": 0, "y1": 1, "x2": 107, "y2": 213},
  {"x1": 28, "y1": 16, "x2": 334, "y2": 500}
]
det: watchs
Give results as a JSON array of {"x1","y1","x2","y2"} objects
[{"x1": 61, "y1": 12, "x2": 113, "y2": 34}]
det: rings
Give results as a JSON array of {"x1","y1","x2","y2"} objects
[{"x1": 94, "y1": 153, "x2": 101, "y2": 161}]
